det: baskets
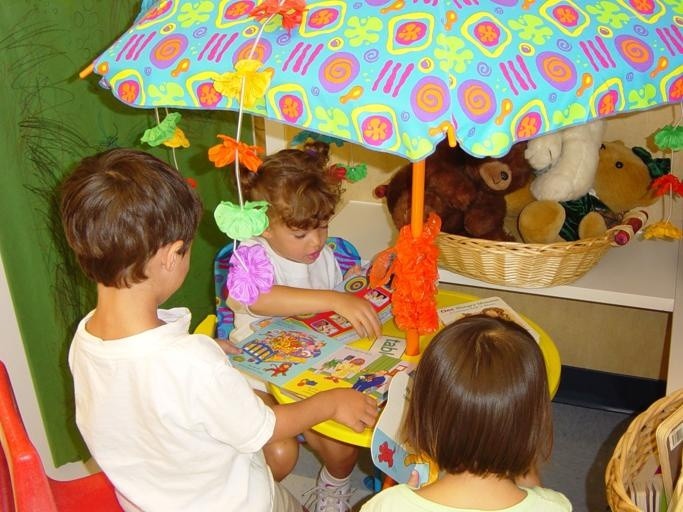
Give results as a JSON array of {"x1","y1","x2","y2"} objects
[
  {"x1": 436, "y1": 209, "x2": 628, "y2": 290},
  {"x1": 602, "y1": 388, "x2": 682, "y2": 512}
]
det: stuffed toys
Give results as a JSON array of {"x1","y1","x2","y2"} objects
[
  {"x1": 384, "y1": 133, "x2": 536, "y2": 250},
  {"x1": 522, "y1": 113, "x2": 607, "y2": 203},
  {"x1": 502, "y1": 137, "x2": 656, "y2": 244}
]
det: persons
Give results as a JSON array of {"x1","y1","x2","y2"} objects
[
  {"x1": 220, "y1": 139, "x2": 384, "y2": 512},
  {"x1": 356, "y1": 313, "x2": 579, "y2": 512},
  {"x1": 55, "y1": 145, "x2": 382, "y2": 512}
]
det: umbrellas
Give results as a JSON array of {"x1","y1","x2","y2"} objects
[{"x1": 70, "y1": 0, "x2": 682, "y2": 210}]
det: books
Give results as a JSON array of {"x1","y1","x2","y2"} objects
[
  {"x1": 435, "y1": 294, "x2": 542, "y2": 346},
  {"x1": 244, "y1": 248, "x2": 411, "y2": 347},
  {"x1": 624, "y1": 400, "x2": 682, "y2": 512},
  {"x1": 223, "y1": 319, "x2": 413, "y2": 413}
]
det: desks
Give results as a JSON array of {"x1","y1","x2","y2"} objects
[
  {"x1": 226, "y1": 280, "x2": 561, "y2": 492},
  {"x1": 326, "y1": 200, "x2": 683, "y2": 406}
]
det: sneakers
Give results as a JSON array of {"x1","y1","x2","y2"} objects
[{"x1": 314, "y1": 476, "x2": 352, "y2": 512}]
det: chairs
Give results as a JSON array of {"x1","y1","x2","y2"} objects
[
  {"x1": 214, "y1": 236, "x2": 361, "y2": 341},
  {"x1": 0, "y1": 360, "x2": 125, "y2": 512}
]
